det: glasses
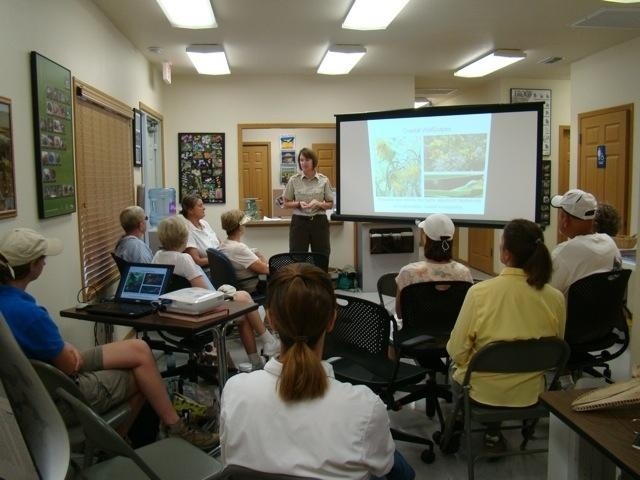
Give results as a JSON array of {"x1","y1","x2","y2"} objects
[{"x1": 145, "y1": 216, "x2": 148, "y2": 220}]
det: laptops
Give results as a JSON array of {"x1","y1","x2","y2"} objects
[{"x1": 88, "y1": 262, "x2": 175, "y2": 319}]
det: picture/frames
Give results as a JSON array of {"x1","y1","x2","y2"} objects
[
  {"x1": 178, "y1": 132, "x2": 226, "y2": 205},
  {"x1": 280, "y1": 136, "x2": 297, "y2": 184},
  {"x1": 0, "y1": 95, "x2": 18, "y2": 219},
  {"x1": 133, "y1": 108, "x2": 142, "y2": 167}
]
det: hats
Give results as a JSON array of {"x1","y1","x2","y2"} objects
[
  {"x1": 417, "y1": 214, "x2": 455, "y2": 242},
  {"x1": 550, "y1": 189, "x2": 597, "y2": 221},
  {"x1": 239, "y1": 213, "x2": 250, "y2": 226},
  {"x1": 0, "y1": 227, "x2": 63, "y2": 279}
]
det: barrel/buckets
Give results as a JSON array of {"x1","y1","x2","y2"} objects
[{"x1": 149, "y1": 188, "x2": 177, "y2": 224}]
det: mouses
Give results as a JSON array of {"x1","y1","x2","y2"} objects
[{"x1": 76, "y1": 303, "x2": 92, "y2": 311}]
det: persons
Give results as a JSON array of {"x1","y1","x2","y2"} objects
[
  {"x1": 394, "y1": 213, "x2": 474, "y2": 385},
  {"x1": 114, "y1": 205, "x2": 154, "y2": 264},
  {"x1": 218, "y1": 262, "x2": 415, "y2": 479},
  {"x1": 547, "y1": 189, "x2": 623, "y2": 388},
  {"x1": 0, "y1": 227, "x2": 221, "y2": 452},
  {"x1": 150, "y1": 215, "x2": 282, "y2": 372},
  {"x1": 179, "y1": 194, "x2": 221, "y2": 282},
  {"x1": 281, "y1": 148, "x2": 334, "y2": 274},
  {"x1": 594, "y1": 202, "x2": 620, "y2": 237},
  {"x1": 217, "y1": 209, "x2": 271, "y2": 338},
  {"x1": 431, "y1": 219, "x2": 565, "y2": 456}
]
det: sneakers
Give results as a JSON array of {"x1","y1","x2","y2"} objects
[
  {"x1": 433, "y1": 430, "x2": 460, "y2": 454},
  {"x1": 165, "y1": 420, "x2": 219, "y2": 450},
  {"x1": 483, "y1": 431, "x2": 507, "y2": 453},
  {"x1": 262, "y1": 337, "x2": 281, "y2": 356},
  {"x1": 560, "y1": 374, "x2": 574, "y2": 388}
]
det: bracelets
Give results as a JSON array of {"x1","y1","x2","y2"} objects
[{"x1": 322, "y1": 199, "x2": 326, "y2": 211}]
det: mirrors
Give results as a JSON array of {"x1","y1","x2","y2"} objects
[{"x1": 238, "y1": 122, "x2": 337, "y2": 219}]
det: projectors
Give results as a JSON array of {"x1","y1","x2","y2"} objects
[{"x1": 157, "y1": 287, "x2": 225, "y2": 315}]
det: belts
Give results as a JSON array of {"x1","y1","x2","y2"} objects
[{"x1": 307, "y1": 215, "x2": 314, "y2": 221}]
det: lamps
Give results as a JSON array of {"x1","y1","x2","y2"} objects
[
  {"x1": 341, "y1": 0, "x2": 410, "y2": 32},
  {"x1": 414, "y1": 97, "x2": 430, "y2": 108},
  {"x1": 453, "y1": 48, "x2": 527, "y2": 80},
  {"x1": 186, "y1": 43, "x2": 233, "y2": 76},
  {"x1": 316, "y1": 45, "x2": 366, "y2": 76},
  {"x1": 156, "y1": 0, "x2": 219, "y2": 31}
]
